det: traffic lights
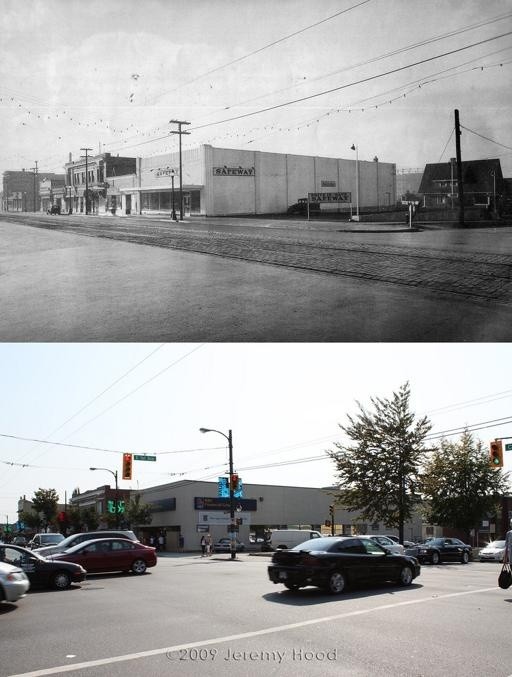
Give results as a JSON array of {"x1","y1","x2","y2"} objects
[
  {"x1": 122, "y1": 452, "x2": 132, "y2": 479},
  {"x1": 232, "y1": 474, "x2": 239, "y2": 490},
  {"x1": 237, "y1": 518, "x2": 242, "y2": 525},
  {"x1": 329, "y1": 503, "x2": 334, "y2": 515},
  {"x1": 489, "y1": 440, "x2": 503, "y2": 468},
  {"x1": 325, "y1": 520, "x2": 332, "y2": 527}
]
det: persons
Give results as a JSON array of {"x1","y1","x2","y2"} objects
[
  {"x1": 503, "y1": 519, "x2": 512, "y2": 572},
  {"x1": 158, "y1": 534, "x2": 165, "y2": 551},
  {"x1": 200, "y1": 536, "x2": 207, "y2": 557},
  {"x1": 206, "y1": 534, "x2": 212, "y2": 557},
  {"x1": 149, "y1": 536, "x2": 154, "y2": 545}
]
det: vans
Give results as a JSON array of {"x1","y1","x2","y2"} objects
[{"x1": 264, "y1": 529, "x2": 323, "y2": 551}]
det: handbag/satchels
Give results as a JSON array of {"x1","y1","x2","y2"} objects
[{"x1": 499, "y1": 562, "x2": 512, "y2": 589}]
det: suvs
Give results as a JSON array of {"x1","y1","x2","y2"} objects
[
  {"x1": 47, "y1": 204, "x2": 61, "y2": 214},
  {"x1": 30, "y1": 529, "x2": 141, "y2": 558}
]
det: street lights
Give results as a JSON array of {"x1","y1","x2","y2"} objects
[
  {"x1": 373, "y1": 156, "x2": 380, "y2": 212},
  {"x1": 90, "y1": 467, "x2": 119, "y2": 530},
  {"x1": 24, "y1": 191, "x2": 27, "y2": 211},
  {"x1": 68, "y1": 184, "x2": 73, "y2": 213},
  {"x1": 199, "y1": 427, "x2": 237, "y2": 558},
  {"x1": 170, "y1": 168, "x2": 177, "y2": 220},
  {"x1": 350, "y1": 143, "x2": 359, "y2": 215}
]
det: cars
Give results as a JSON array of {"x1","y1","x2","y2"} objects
[
  {"x1": 214, "y1": 536, "x2": 244, "y2": 552},
  {"x1": 249, "y1": 536, "x2": 264, "y2": 544},
  {"x1": 12, "y1": 536, "x2": 27, "y2": 547},
  {"x1": 30, "y1": 532, "x2": 66, "y2": 549},
  {"x1": 360, "y1": 534, "x2": 442, "y2": 564},
  {"x1": 44, "y1": 537, "x2": 159, "y2": 575},
  {"x1": 267, "y1": 536, "x2": 421, "y2": 595},
  {"x1": 0, "y1": 544, "x2": 87, "y2": 590},
  {"x1": 402, "y1": 536, "x2": 472, "y2": 564},
  {"x1": 0, "y1": 562, "x2": 30, "y2": 604},
  {"x1": 479, "y1": 540, "x2": 509, "y2": 564},
  {"x1": 290, "y1": 198, "x2": 322, "y2": 217}
]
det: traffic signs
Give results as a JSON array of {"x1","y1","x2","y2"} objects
[
  {"x1": 504, "y1": 443, "x2": 512, "y2": 451},
  {"x1": 134, "y1": 454, "x2": 157, "y2": 461}
]
